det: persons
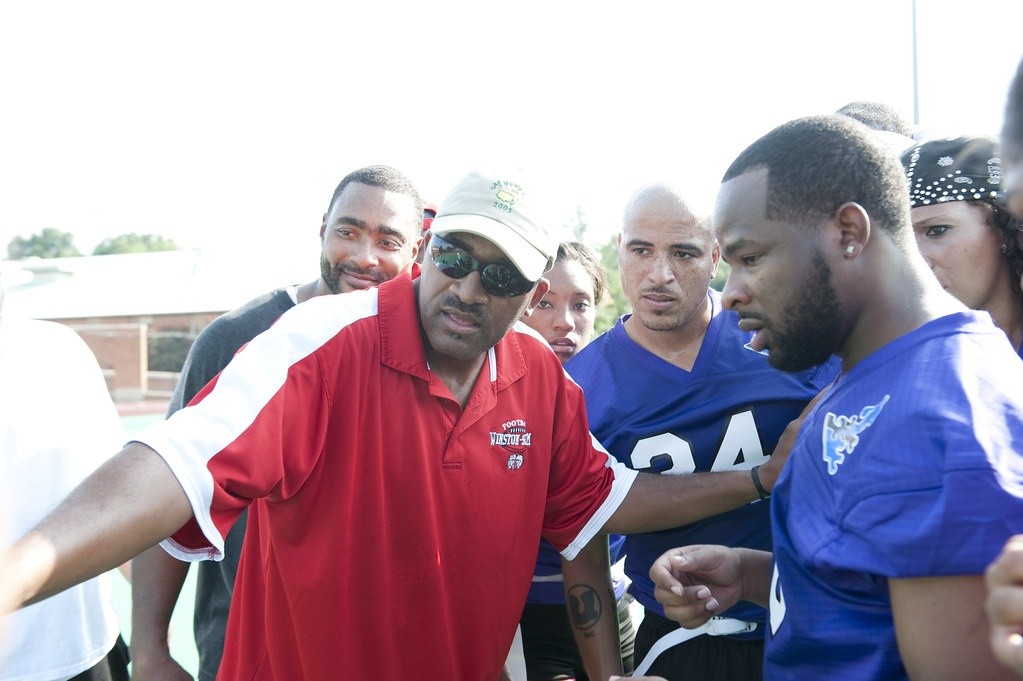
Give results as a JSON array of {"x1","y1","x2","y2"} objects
[
  {"x1": 4, "y1": 321, "x2": 133, "y2": 680},
  {"x1": 649, "y1": 117, "x2": 1023, "y2": 680},
  {"x1": 517, "y1": 242, "x2": 647, "y2": 679},
  {"x1": 561, "y1": 180, "x2": 845, "y2": 681},
  {"x1": 3, "y1": 167, "x2": 836, "y2": 680},
  {"x1": 897, "y1": 81, "x2": 1023, "y2": 681},
  {"x1": 128, "y1": 169, "x2": 512, "y2": 681}
]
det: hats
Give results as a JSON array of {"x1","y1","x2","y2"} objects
[{"x1": 429, "y1": 159, "x2": 565, "y2": 280}]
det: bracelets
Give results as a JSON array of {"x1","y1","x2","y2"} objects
[{"x1": 752, "y1": 465, "x2": 771, "y2": 501}]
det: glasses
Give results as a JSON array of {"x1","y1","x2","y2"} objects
[{"x1": 428, "y1": 232, "x2": 535, "y2": 299}]
